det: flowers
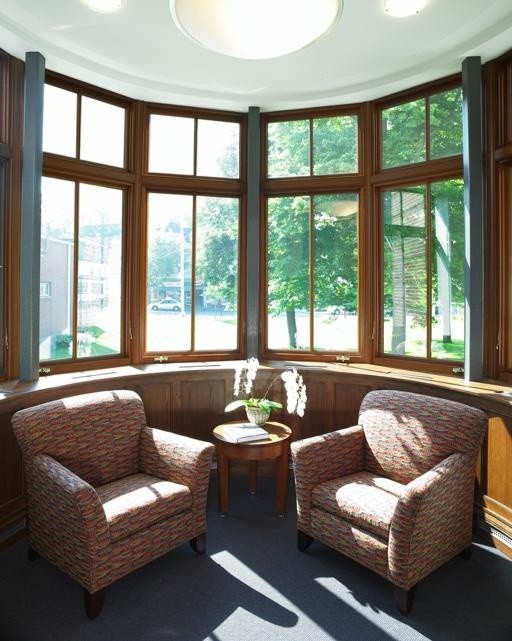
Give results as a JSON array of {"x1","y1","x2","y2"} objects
[{"x1": 223, "y1": 357, "x2": 308, "y2": 417}]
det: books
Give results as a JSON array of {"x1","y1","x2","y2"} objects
[{"x1": 220, "y1": 423, "x2": 268, "y2": 444}]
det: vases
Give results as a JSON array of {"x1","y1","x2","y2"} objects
[{"x1": 244, "y1": 405, "x2": 271, "y2": 426}]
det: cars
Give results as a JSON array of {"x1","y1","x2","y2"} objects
[
  {"x1": 326, "y1": 301, "x2": 357, "y2": 315},
  {"x1": 147, "y1": 296, "x2": 181, "y2": 312}
]
